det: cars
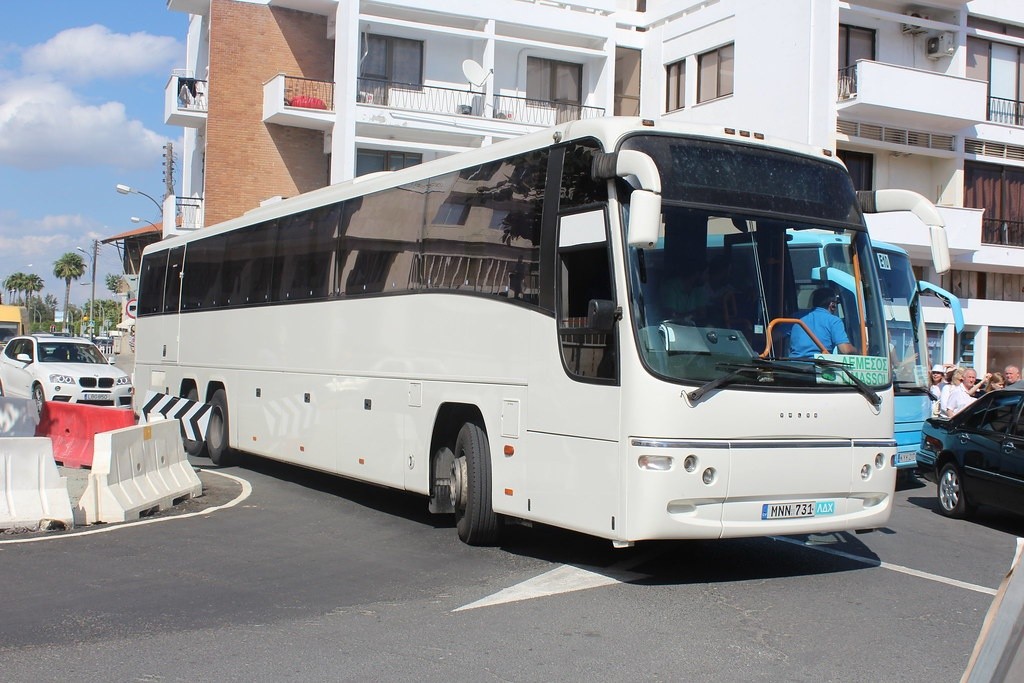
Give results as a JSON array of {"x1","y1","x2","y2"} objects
[
  {"x1": 916, "y1": 381, "x2": 1024, "y2": 524},
  {"x1": 0, "y1": 335, "x2": 133, "y2": 410}
]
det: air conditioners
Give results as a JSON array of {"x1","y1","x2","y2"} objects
[
  {"x1": 901, "y1": 11, "x2": 930, "y2": 36},
  {"x1": 493, "y1": 109, "x2": 514, "y2": 121},
  {"x1": 895, "y1": 132, "x2": 918, "y2": 156},
  {"x1": 357, "y1": 91, "x2": 373, "y2": 104},
  {"x1": 925, "y1": 32, "x2": 954, "y2": 60}
]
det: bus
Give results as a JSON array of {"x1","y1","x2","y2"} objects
[
  {"x1": 130, "y1": 116, "x2": 949, "y2": 550},
  {"x1": 650, "y1": 230, "x2": 965, "y2": 471}
]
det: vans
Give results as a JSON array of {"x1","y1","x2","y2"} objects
[{"x1": 0, "y1": 304, "x2": 30, "y2": 346}]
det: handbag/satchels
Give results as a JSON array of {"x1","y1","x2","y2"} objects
[{"x1": 932, "y1": 399, "x2": 941, "y2": 415}]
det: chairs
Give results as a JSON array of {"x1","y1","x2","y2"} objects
[
  {"x1": 53, "y1": 349, "x2": 66, "y2": 360},
  {"x1": 28, "y1": 348, "x2": 48, "y2": 360}
]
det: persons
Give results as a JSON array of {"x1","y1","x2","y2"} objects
[
  {"x1": 886, "y1": 328, "x2": 918, "y2": 378},
  {"x1": 646, "y1": 251, "x2": 782, "y2": 363},
  {"x1": 929, "y1": 361, "x2": 1024, "y2": 420},
  {"x1": 787, "y1": 288, "x2": 856, "y2": 355}
]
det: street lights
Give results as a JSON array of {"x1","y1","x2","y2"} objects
[
  {"x1": 76, "y1": 246, "x2": 99, "y2": 342},
  {"x1": 3, "y1": 264, "x2": 33, "y2": 305}
]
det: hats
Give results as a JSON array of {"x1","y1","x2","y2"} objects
[
  {"x1": 946, "y1": 364, "x2": 957, "y2": 373},
  {"x1": 931, "y1": 364, "x2": 944, "y2": 374}
]
